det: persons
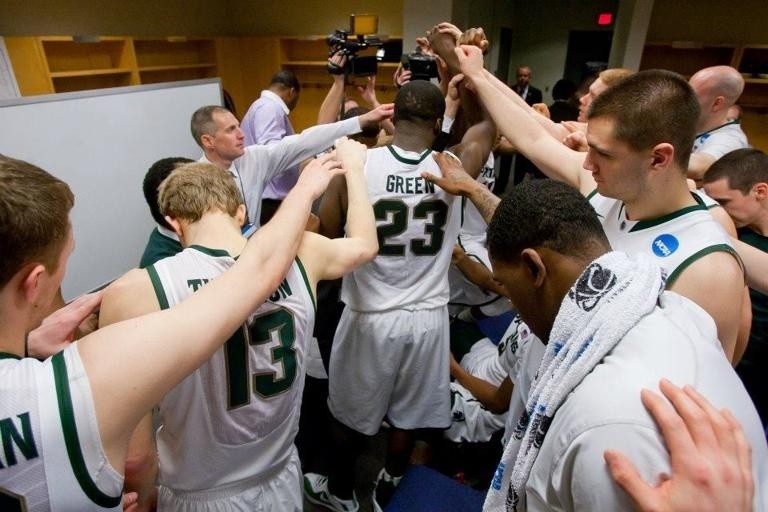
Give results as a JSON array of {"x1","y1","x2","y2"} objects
[{"x1": 1, "y1": 20, "x2": 768, "y2": 511}]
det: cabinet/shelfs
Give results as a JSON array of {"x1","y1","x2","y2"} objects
[
  {"x1": 639, "y1": 41, "x2": 768, "y2": 108},
  {"x1": 275, "y1": 35, "x2": 402, "y2": 133},
  {"x1": 1, "y1": 35, "x2": 222, "y2": 96}
]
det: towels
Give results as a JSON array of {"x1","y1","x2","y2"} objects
[{"x1": 482, "y1": 248, "x2": 668, "y2": 512}]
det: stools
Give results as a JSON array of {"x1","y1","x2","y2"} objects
[{"x1": 384, "y1": 465, "x2": 486, "y2": 511}]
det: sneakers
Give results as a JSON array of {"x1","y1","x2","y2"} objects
[
  {"x1": 372, "y1": 468, "x2": 403, "y2": 512},
  {"x1": 303, "y1": 472, "x2": 359, "y2": 512}
]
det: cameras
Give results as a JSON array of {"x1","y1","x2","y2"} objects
[
  {"x1": 325, "y1": 29, "x2": 381, "y2": 78},
  {"x1": 400, "y1": 52, "x2": 440, "y2": 80}
]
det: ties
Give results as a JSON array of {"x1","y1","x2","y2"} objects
[{"x1": 517, "y1": 89, "x2": 524, "y2": 97}]
